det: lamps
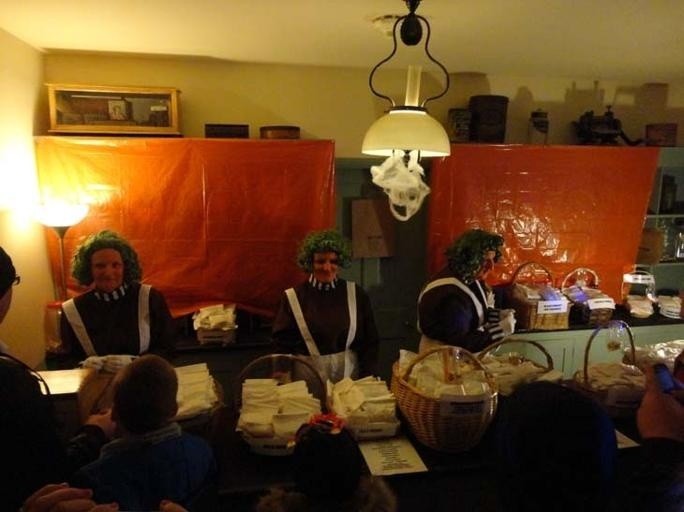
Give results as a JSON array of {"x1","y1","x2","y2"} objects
[{"x1": 360, "y1": 0, "x2": 450, "y2": 223}]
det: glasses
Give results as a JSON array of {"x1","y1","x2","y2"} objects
[{"x1": 10, "y1": 274, "x2": 20, "y2": 287}]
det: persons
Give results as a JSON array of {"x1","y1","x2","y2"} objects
[
  {"x1": 23, "y1": 354, "x2": 222, "y2": 512},
  {"x1": 61, "y1": 230, "x2": 176, "y2": 362},
  {"x1": 477, "y1": 348, "x2": 684, "y2": 512},
  {"x1": 255, "y1": 413, "x2": 396, "y2": 512},
  {"x1": 272, "y1": 230, "x2": 379, "y2": 381},
  {"x1": 0, "y1": 246, "x2": 56, "y2": 511},
  {"x1": 417, "y1": 230, "x2": 517, "y2": 356}
]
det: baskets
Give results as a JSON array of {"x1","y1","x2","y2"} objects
[
  {"x1": 510, "y1": 261, "x2": 656, "y2": 330},
  {"x1": 234, "y1": 354, "x2": 332, "y2": 457},
  {"x1": 393, "y1": 321, "x2": 645, "y2": 454},
  {"x1": 170, "y1": 367, "x2": 225, "y2": 425}
]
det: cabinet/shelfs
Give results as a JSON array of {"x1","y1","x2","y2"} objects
[
  {"x1": 628, "y1": 145, "x2": 684, "y2": 294},
  {"x1": 41, "y1": 83, "x2": 183, "y2": 137}
]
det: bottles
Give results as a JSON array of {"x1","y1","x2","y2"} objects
[{"x1": 526, "y1": 106, "x2": 549, "y2": 145}]
